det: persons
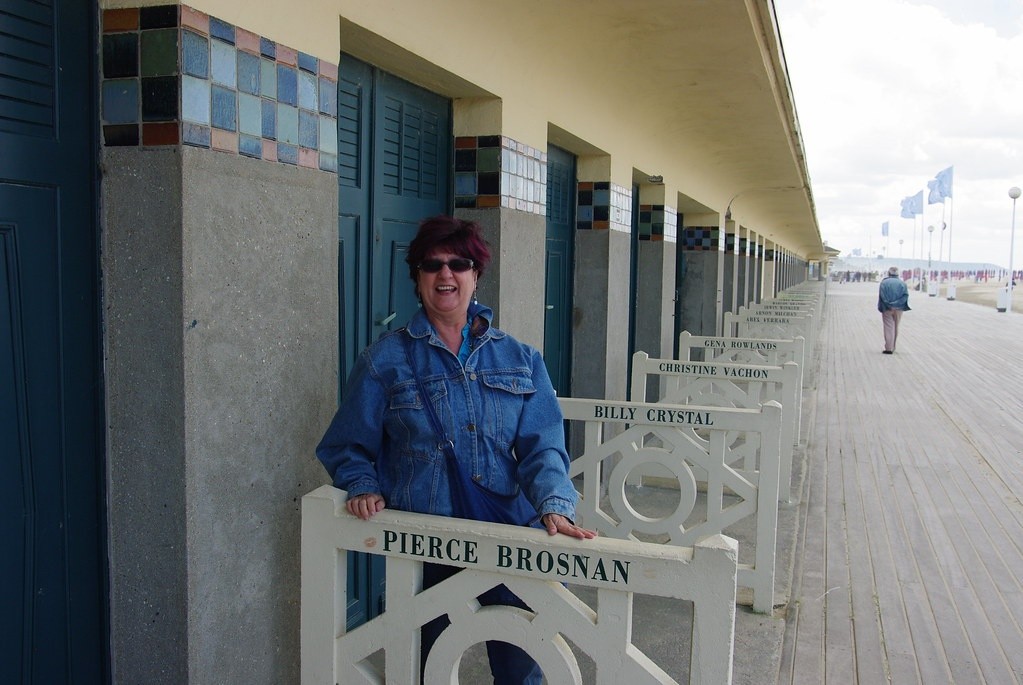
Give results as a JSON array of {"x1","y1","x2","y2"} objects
[
  {"x1": 315, "y1": 215, "x2": 597, "y2": 685},
  {"x1": 878, "y1": 267, "x2": 911, "y2": 354},
  {"x1": 1006, "y1": 278, "x2": 1016, "y2": 292},
  {"x1": 839, "y1": 269, "x2": 871, "y2": 284}
]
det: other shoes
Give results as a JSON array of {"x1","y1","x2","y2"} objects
[{"x1": 883, "y1": 350, "x2": 892, "y2": 354}]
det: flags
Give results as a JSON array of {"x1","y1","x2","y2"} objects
[
  {"x1": 882, "y1": 221, "x2": 889, "y2": 237},
  {"x1": 900, "y1": 190, "x2": 923, "y2": 219},
  {"x1": 926, "y1": 166, "x2": 953, "y2": 204}
]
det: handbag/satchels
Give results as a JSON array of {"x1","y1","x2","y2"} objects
[{"x1": 453, "y1": 481, "x2": 548, "y2": 527}]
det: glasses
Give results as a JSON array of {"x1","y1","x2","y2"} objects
[{"x1": 416, "y1": 258, "x2": 475, "y2": 272}]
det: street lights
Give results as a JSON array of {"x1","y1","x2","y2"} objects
[
  {"x1": 927, "y1": 226, "x2": 934, "y2": 283},
  {"x1": 1006, "y1": 187, "x2": 1021, "y2": 314},
  {"x1": 899, "y1": 239, "x2": 902, "y2": 276}
]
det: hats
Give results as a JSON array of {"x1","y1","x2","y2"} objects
[{"x1": 889, "y1": 266, "x2": 898, "y2": 275}]
